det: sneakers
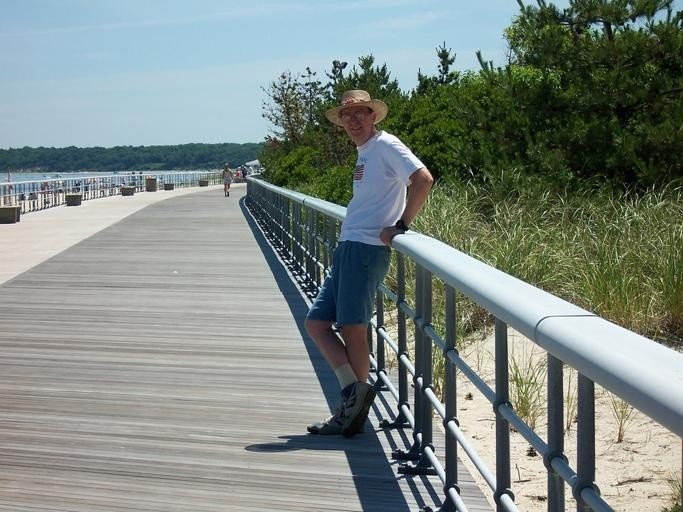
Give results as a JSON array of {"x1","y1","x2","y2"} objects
[
  {"x1": 340, "y1": 380, "x2": 377, "y2": 439},
  {"x1": 306, "y1": 407, "x2": 365, "y2": 435}
]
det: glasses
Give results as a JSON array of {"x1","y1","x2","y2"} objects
[{"x1": 338, "y1": 109, "x2": 370, "y2": 123}]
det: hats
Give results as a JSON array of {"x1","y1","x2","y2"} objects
[{"x1": 325, "y1": 89, "x2": 389, "y2": 127}]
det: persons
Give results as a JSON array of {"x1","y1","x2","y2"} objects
[
  {"x1": 235, "y1": 164, "x2": 264, "y2": 183},
  {"x1": 301, "y1": 88, "x2": 437, "y2": 437},
  {"x1": 221, "y1": 163, "x2": 233, "y2": 197},
  {"x1": 15, "y1": 169, "x2": 143, "y2": 202}
]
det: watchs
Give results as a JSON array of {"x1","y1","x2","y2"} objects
[{"x1": 395, "y1": 220, "x2": 409, "y2": 232}]
